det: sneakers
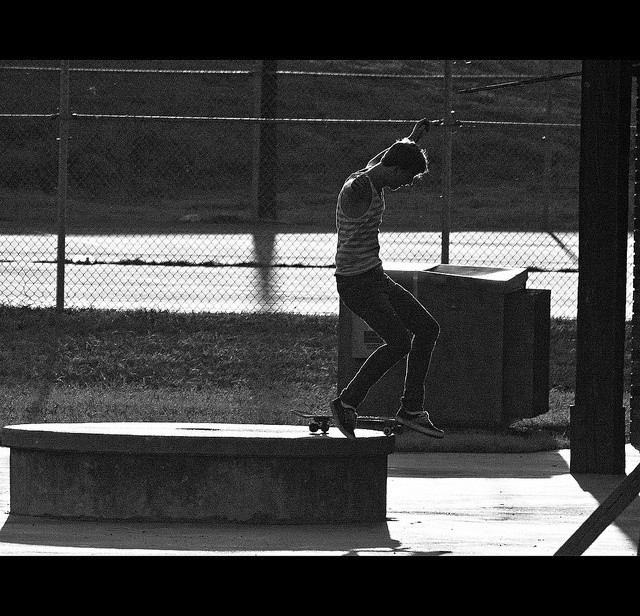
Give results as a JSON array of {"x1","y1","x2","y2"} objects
[
  {"x1": 330, "y1": 397, "x2": 356, "y2": 437},
  {"x1": 393, "y1": 407, "x2": 444, "y2": 438}
]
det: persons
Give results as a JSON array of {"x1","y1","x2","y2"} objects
[{"x1": 328, "y1": 117, "x2": 445, "y2": 440}]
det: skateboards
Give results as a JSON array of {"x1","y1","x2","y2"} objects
[{"x1": 293, "y1": 406, "x2": 402, "y2": 435}]
